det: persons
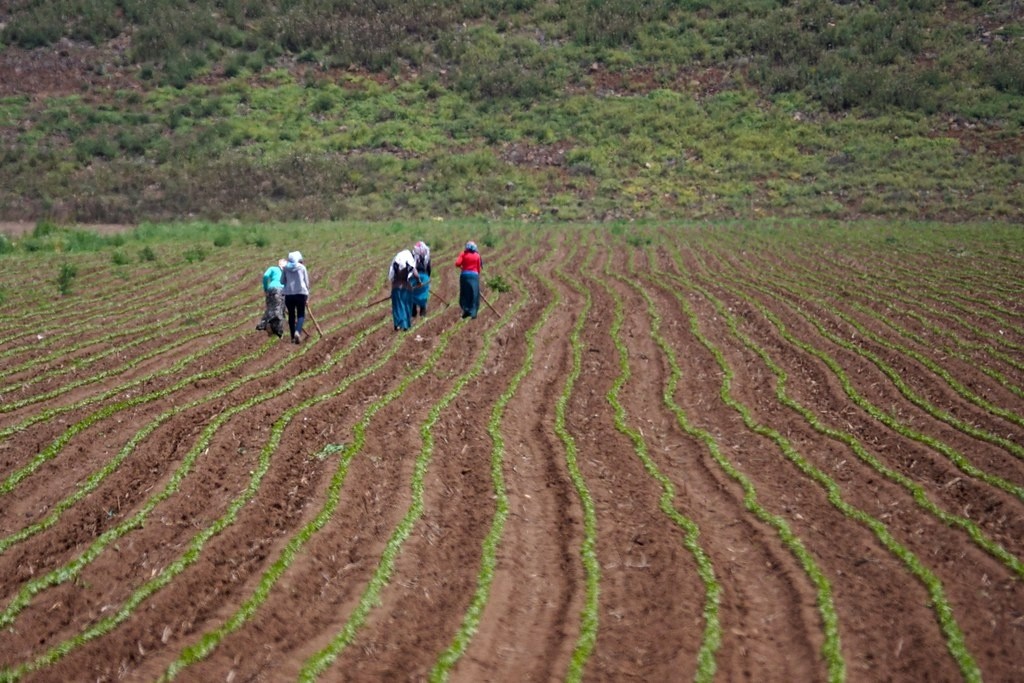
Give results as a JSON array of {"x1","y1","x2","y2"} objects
[
  {"x1": 411, "y1": 240, "x2": 432, "y2": 317},
  {"x1": 388, "y1": 249, "x2": 423, "y2": 331},
  {"x1": 455, "y1": 241, "x2": 484, "y2": 320},
  {"x1": 280, "y1": 251, "x2": 309, "y2": 344},
  {"x1": 261, "y1": 258, "x2": 289, "y2": 340}
]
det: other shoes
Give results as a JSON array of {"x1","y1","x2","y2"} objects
[
  {"x1": 462, "y1": 310, "x2": 469, "y2": 318},
  {"x1": 291, "y1": 339, "x2": 295, "y2": 343},
  {"x1": 295, "y1": 332, "x2": 300, "y2": 344}
]
